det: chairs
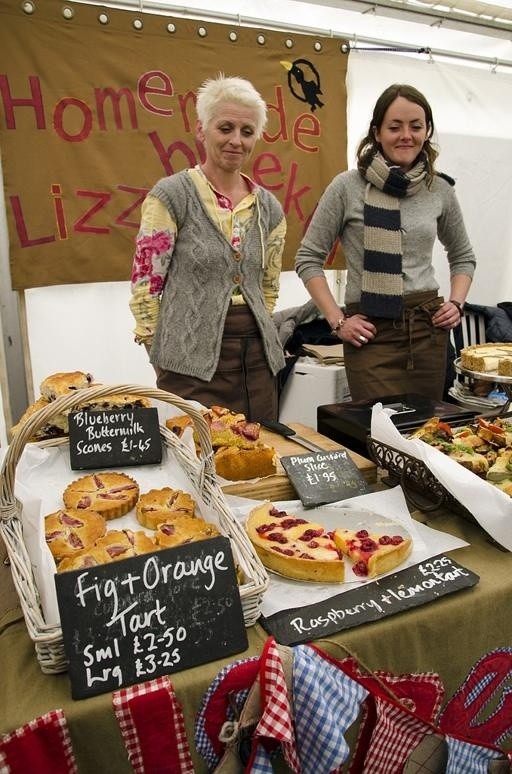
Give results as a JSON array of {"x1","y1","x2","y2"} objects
[{"x1": 446, "y1": 311, "x2": 486, "y2": 388}]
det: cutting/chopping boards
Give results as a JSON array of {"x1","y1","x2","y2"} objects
[{"x1": 218, "y1": 423, "x2": 378, "y2": 501}]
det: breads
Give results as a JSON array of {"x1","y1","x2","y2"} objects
[{"x1": 408, "y1": 416, "x2": 512, "y2": 497}]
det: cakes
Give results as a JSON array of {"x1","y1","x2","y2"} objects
[
  {"x1": 136, "y1": 487, "x2": 195, "y2": 530},
  {"x1": 7, "y1": 370, "x2": 150, "y2": 443},
  {"x1": 328, "y1": 527, "x2": 411, "y2": 577},
  {"x1": 58, "y1": 546, "x2": 118, "y2": 573},
  {"x1": 151, "y1": 516, "x2": 218, "y2": 549},
  {"x1": 97, "y1": 529, "x2": 158, "y2": 558},
  {"x1": 460, "y1": 341, "x2": 512, "y2": 376},
  {"x1": 244, "y1": 499, "x2": 345, "y2": 584},
  {"x1": 167, "y1": 405, "x2": 278, "y2": 480},
  {"x1": 42, "y1": 510, "x2": 106, "y2": 561},
  {"x1": 62, "y1": 472, "x2": 139, "y2": 520}
]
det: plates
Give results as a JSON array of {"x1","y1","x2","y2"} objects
[{"x1": 242, "y1": 504, "x2": 415, "y2": 588}]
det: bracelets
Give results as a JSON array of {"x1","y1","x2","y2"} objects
[
  {"x1": 331, "y1": 313, "x2": 348, "y2": 337},
  {"x1": 448, "y1": 299, "x2": 464, "y2": 317}
]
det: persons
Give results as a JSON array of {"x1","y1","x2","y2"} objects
[
  {"x1": 297, "y1": 85, "x2": 476, "y2": 402},
  {"x1": 130, "y1": 72, "x2": 288, "y2": 424}
]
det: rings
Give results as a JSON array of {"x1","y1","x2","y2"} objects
[{"x1": 357, "y1": 335, "x2": 365, "y2": 342}]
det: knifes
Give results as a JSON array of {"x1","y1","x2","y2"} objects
[{"x1": 258, "y1": 413, "x2": 327, "y2": 455}]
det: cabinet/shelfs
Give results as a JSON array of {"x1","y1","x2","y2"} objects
[{"x1": 278, "y1": 360, "x2": 351, "y2": 433}]
